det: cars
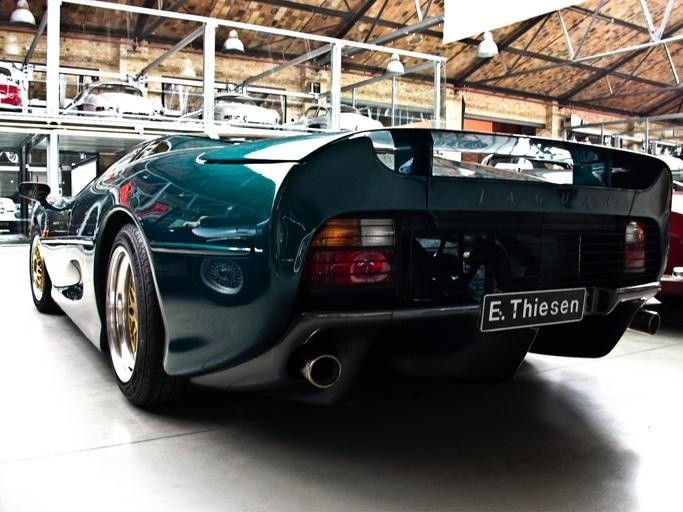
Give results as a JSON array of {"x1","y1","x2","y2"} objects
[
  {"x1": 300, "y1": 103, "x2": 384, "y2": 133},
  {"x1": 0, "y1": 62, "x2": 29, "y2": 113},
  {"x1": 79, "y1": 81, "x2": 165, "y2": 119},
  {"x1": 197, "y1": 92, "x2": 281, "y2": 124}
]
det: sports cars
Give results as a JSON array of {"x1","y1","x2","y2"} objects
[{"x1": 19, "y1": 129, "x2": 673, "y2": 408}]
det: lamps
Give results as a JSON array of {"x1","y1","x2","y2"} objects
[
  {"x1": 477, "y1": 31, "x2": 498, "y2": 58},
  {"x1": 9, "y1": 0, "x2": 37, "y2": 27},
  {"x1": 386, "y1": 52, "x2": 404, "y2": 76},
  {"x1": 223, "y1": 27, "x2": 245, "y2": 54}
]
